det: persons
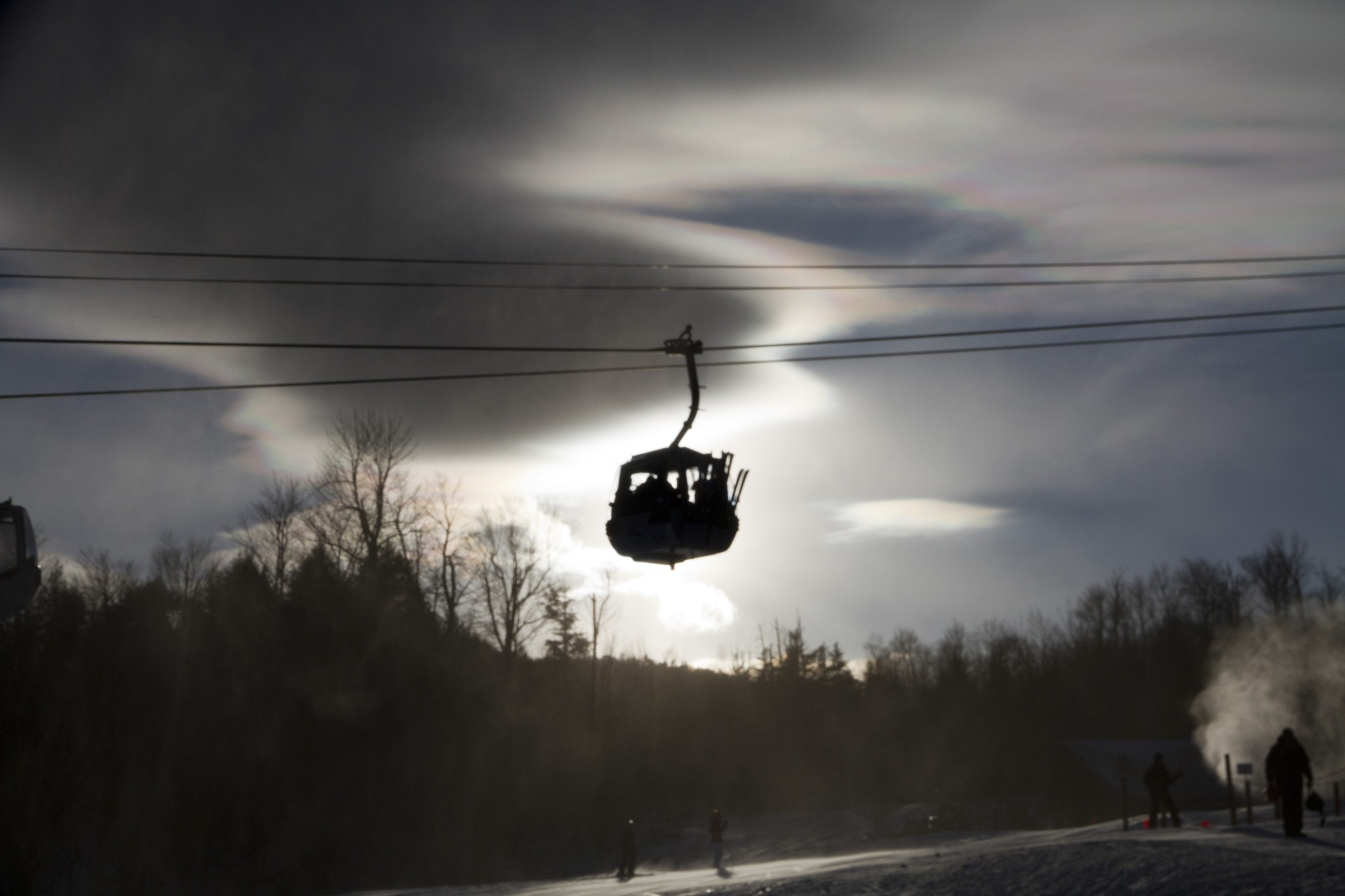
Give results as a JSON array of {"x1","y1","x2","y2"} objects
[
  {"x1": 1144, "y1": 753, "x2": 1182, "y2": 828},
  {"x1": 617, "y1": 818, "x2": 638, "y2": 878},
  {"x1": 1266, "y1": 727, "x2": 1314, "y2": 838},
  {"x1": 710, "y1": 810, "x2": 728, "y2": 869}
]
